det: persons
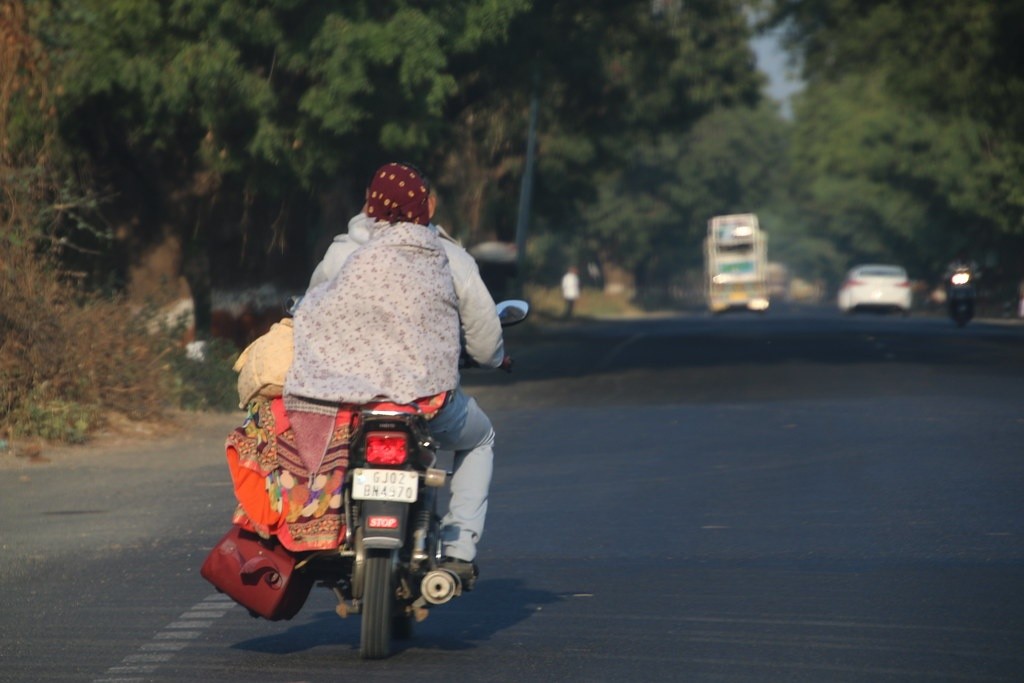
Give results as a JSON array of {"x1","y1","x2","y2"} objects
[
  {"x1": 560, "y1": 267, "x2": 579, "y2": 316},
  {"x1": 282, "y1": 162, "x2": 461, "y2": 475},
  {"x1": 304, "y1": 162, "x2": 510, "y2": 578}
]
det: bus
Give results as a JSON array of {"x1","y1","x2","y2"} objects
[{"x1": 705, "y1": 214, "x2": 770, "y2": 313}]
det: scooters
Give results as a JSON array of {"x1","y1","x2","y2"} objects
[{"x1": 944, "y1": 263, "x2": 979, "y2": 328}]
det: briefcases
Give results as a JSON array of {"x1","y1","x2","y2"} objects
[{"x1": 199, "y1": 525, "x2": 322, "y2": 621}]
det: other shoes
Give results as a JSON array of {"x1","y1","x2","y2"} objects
[{"x1": 445, "y1": 556, "x2": 478, "y2": 580}]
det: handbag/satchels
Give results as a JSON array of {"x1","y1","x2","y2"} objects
[{"x1": 232, "y1": 317, "x2": 295, "y2": 409}]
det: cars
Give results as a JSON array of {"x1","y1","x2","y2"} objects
[{"x1": 839, "y1": 265, "x2": 912, "y2": 317}]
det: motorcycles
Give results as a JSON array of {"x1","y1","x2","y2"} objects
[{"x1": 284, "y1": 293, "x2": 530, "y2": 657}]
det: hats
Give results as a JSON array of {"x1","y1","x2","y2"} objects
[{"x1": 368, "y1": 161, "x2": 429, "y2": 226}]
